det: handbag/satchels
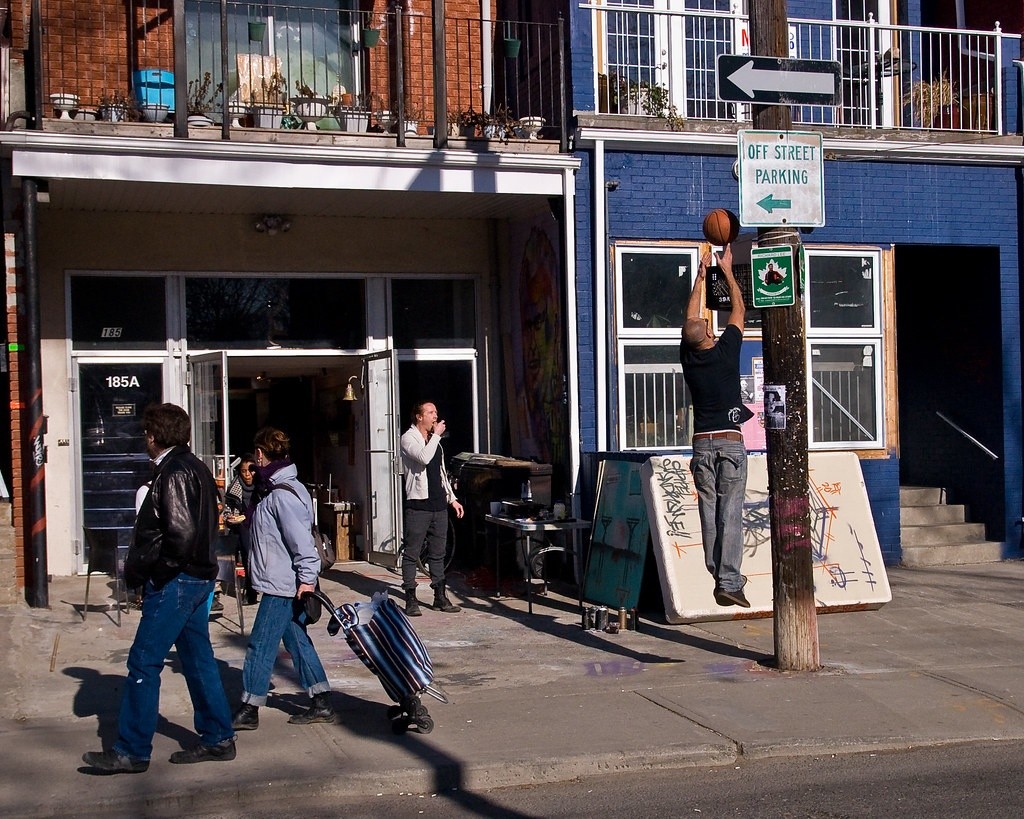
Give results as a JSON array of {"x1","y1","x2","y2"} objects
[
  {"x1": 301, "y1": 576, "x2": 323, "y2": 625},
  {"x1": 311, "y1": 528, "x2": 334, "y2": 573}
]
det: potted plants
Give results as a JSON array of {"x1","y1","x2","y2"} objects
[
  {"x1": 95, "y1": 72, "x2": 527, "y2": 141},
  {"x1": 901, "y1": 73, "x2": 962, "y2": 129},
  {"x1": 609, "y1": 73, "x2": 686, "y2": 130}
]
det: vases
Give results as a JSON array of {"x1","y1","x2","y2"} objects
[
  {"x1": 520, "y1": 116, "x2": 547, "y2": 141},
  {"x1": 226, "y1": 101, "x2": 248, "y2": 128},
  {"x1": 249, "y1": 21, "x2": 265, "y2": 41},
  {"x1": 49, "y1": 94, "x2": 82, "y2": 121},
  {"x1": 363, "y1": 28, "x2": 382, "y2": 48},
  {"x1": 962, "y1": 92, "x2": 994, "y2": 132},
  {"x1": 505, "y1": 38, "x2": 521, "y2": 58},
  {"x1": 141, "y1": 103, "x2": 169, "y2": 123}
]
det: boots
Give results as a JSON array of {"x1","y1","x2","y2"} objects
[
  {"x1": 433, "y1": 582, "x2": 462, "y2": 612},
  {"x1": 230, "y1": 702, "x2": 259, "y2": 730},
  {"x1": 288, "y1": 693, "x2": 335, "y2": 724},
  {"x1": 406, "y1": 588, "x2": 422, "y2": 616}
]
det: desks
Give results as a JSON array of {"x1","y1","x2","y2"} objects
[{"x1": 484, "y1": 512, "x2": 591, "y2": 615}]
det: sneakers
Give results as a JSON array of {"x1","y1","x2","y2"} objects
[
  {"x1": 82, "y1": 751, "x2": 149, "y2": 771},
  {"x1": 171, "y1": 734, "x2": 237, "y2": 763}
]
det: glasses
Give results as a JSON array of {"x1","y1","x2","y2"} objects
[{"x1": 704, "y1": 319, "x2": 711, "y2": 338}]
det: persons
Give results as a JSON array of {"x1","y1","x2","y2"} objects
[
  {"x1": 81, "y1": 403, "x2": 237, "y2": 772},
  {"x1": 680, "y1": 242, "x2": 754, "y2": 608},
  {"x1": 231, "y1": 426, "x2": 339, "y2": 729},
  {"x1": 400, "y1": 402, "x2": 464, "y2": 616},
  {"x1": 135, "y1": 452, "x2": 261, "y2": 614},
  {"x1": 765, "y1": 264, "x2": 783, "y2": 286}
]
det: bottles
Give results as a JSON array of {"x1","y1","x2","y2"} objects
[{"x1": 553, "y1": 500, "x2": 566, "y2": 520}]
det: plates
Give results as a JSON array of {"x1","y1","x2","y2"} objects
[{"x1": 230, "y1": 518, "x2": 246, "y2": 524}]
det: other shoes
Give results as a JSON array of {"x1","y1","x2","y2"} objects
[
  {"x1": 719, "y1": 587, "x2": 750, "y2": 609},
  {"x1": 715, "y1": 587, "x2": 734, "y2": 606}
]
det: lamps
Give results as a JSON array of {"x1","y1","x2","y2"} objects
[
  {"x1": 343, "y1": 375, "x2": 363, "y2": 402},
  {"x1": 252, "y1": 215, "x2": 291, "y2": 235},
  {"x1": 252, "y1": 372, "x2": 279, "y2": 390}
]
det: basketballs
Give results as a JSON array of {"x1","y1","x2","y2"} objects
[{"x1": 703, "y1": 209, "x2": 739, "y2": 246}]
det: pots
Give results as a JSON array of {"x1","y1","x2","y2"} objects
[{"x1": 502, "y1": 501, "x2": 544, "y2": 518}]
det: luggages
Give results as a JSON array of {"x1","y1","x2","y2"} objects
[{"x1": 307, "y1": 588, "x2": 448, "y2": 734}]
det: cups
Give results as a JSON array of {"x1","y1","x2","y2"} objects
[{"x1": 490, "y1": 502, "x2": 502, "y2": 517}]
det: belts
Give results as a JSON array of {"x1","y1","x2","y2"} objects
[{"x1": 693, "y1": 432, "x2": 727, "y2": 439}]
